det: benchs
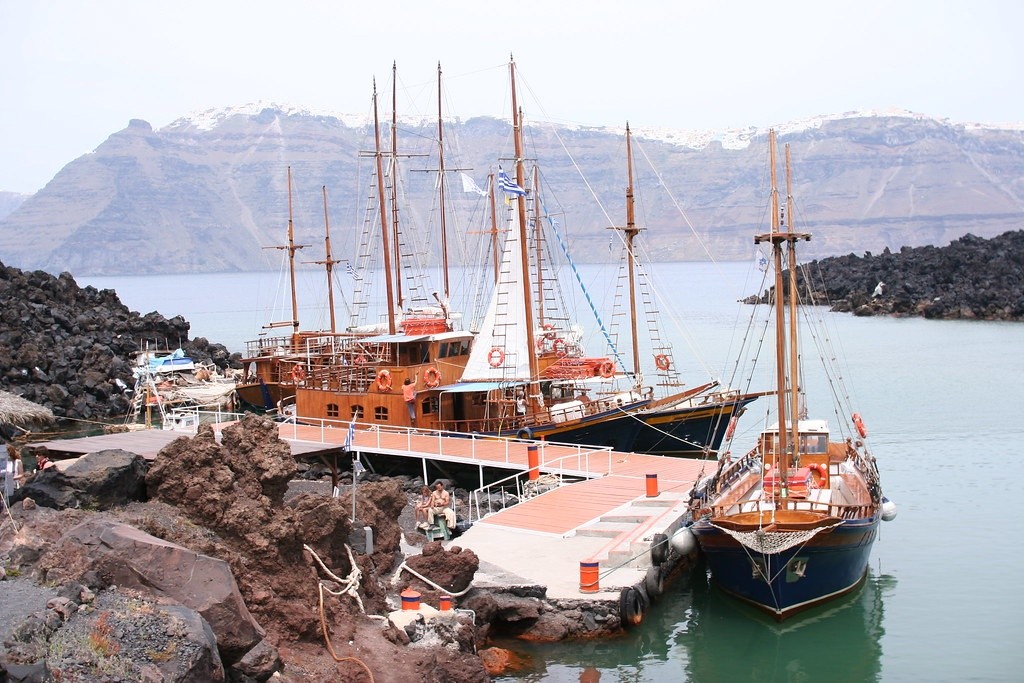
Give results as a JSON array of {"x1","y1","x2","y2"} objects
[{"x1": 424, "y1": 513, "x2": 450, "y2": 545}]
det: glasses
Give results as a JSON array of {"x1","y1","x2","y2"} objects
[{"x1": 35, "y1": 453, "x2": 42, "y2": 456}]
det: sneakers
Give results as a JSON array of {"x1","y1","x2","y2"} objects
[
  {"x1": 446, "y1": 528, "x2": 452, "y2": 535},
  {"x1": 414, "y1": 522, "x2": 421, "y2": 530},
  {"x1": 428, "y1": 524, "x2": 435, "y2": 531},
  {"x1": 419, "y1": 523, "x2": 428, "y2": 528}
]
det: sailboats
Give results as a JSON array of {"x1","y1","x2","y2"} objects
[
  {"x1": 686, "y1": 127, "x2": 884, "y2": 615},
  {"x1": 271, "y1": 52, "x2": 719, "y2": 458},
  {"x1": 608, "y1": 115, "x2": 761, "y2": 456},
  {"x1": 232, "y1": 164, "x2": 346, "y2": 407}
]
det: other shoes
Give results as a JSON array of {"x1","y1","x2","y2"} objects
[{"x1": 411, "y1": 420, "x2": 414, "y2": 422}]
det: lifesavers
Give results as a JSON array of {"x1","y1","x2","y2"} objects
[
  {"x1": 854, "y1": 412, "x2": 867, "y2": 439},
  {"x1": 807, "y1": 463, "x2": 826, "y2": 489},
  {"x1": 653, "y1": 532, "x2": 669, "y2": 563},
  {"x1": 424, "y1": 367, "x2": 440, "y2": 387},
  {"x1": 537, "y1": 338, "x2": 545, "y2": 350},
  {"x1": 646, "y1": 567, "x2": 663, "y2": 597},
  {"x1": 655, "y1": 354, "x2": 670, "y2": 370},
  {"x1": 544, "y1": 324, "x2": 556, "y2": 341},
  {"x1": 553, "y1": 339, "x2": 569, "y2": 357},
  {"x1": 487, "y1": 347, "x2": 505, "y2": 367},
  {"x1": 620, "y1": 587, "x2": 643, "y2": 628},
  {"x1": 377, "y1": 369, "x2": 392, "y2": 391},
  {"x1": 292, "y1": 365, "x2": 303, "y2": 382},
  {"x1": 517, "y1": 428, "x2": 532, "y2": 443},
  {"x1": 726, "y1": 416, "x2": 736, "y2": 441},
  {"x1": 600, "y1": 360, "x2": 616, "y2": 378}
]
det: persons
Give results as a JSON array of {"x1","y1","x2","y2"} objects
[
  {"x1": 6, "y1": 444, "x2": 54, "y2": 489},
  {"x1": 414, "y1": 481, "x2": 454, "y2": 535},
  {"x1": 401, "y1": 370, "x2": 419, "y2": 422},
  {"x1": 516, "y1": 391, "x2": 529, "y2": 427}
]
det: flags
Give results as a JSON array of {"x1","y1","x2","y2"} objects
[
  {"x1": 342, "y1": 423, "x2": 354, "y2": 451},
  {"x1": 498, "y1": 164, "x2": 527, "y2": 196},
  {"x1": 461, "y1": 172, "x2": 486, "y2": 197},
  {"x1": 754, "y1": 246, "x2": 770, "y2": 274},
  {"x1": 608, "y1": 232, "x2": 614, "y2": 254}
]
